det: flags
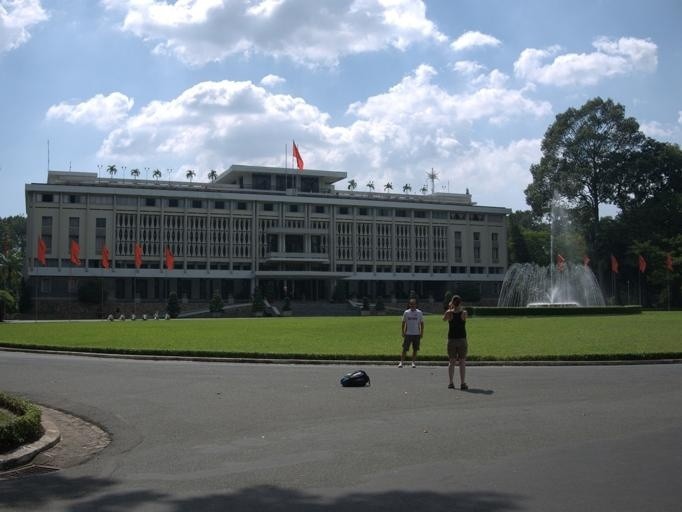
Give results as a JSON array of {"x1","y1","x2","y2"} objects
[
  {"x1": 557, "y1": 254, "x2": 565, "y2": 273},
  {"x1": 37, "y1": 236, "x2": 47, "y2": 266},
  {"x1": 293, "y1": 140, "x2": 303, "y2": 173},
  {"x1": 582, "y1": 254, "x2": 590, "y2": 272},
  {"x1": 102, "y1": 245, "x2": 110, "y2": 273},
  {"x1": 640, "y1": 255, "x2": 647, "y2": 273},
  {"x1": 610, "y1": 253, "x2": 619, "y2": 275},
  {"x1": 135, "y1": 244, "x2": 142, "y2": 269},
  {"x1": 70, "y1": 238, "x2": 80, "y2": 266},
  {"x1": 664, "y1": 256, "x2": 674, "y2": 273},
  {"x1": 164, "y1": 246, "x2": 174, "y2": 272}
]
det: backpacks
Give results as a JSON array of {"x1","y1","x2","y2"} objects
[{"x1": 340, "y1": 369, "x2": 371, "y2": 388}]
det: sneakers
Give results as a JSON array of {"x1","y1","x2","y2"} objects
[
  {"x1": 398, "y1": 363, "x2": 416, "y2": 368},
  {"x1": 448, "y1": 383, "x2": 468, "y2": 390}
]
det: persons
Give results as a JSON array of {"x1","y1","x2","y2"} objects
[
  {"x1": 399, "y1": 297, "x2": 424, "y2": 368},
  {"x1": 442, "y1": 294, "x2": 468, "y2": 389}
]
